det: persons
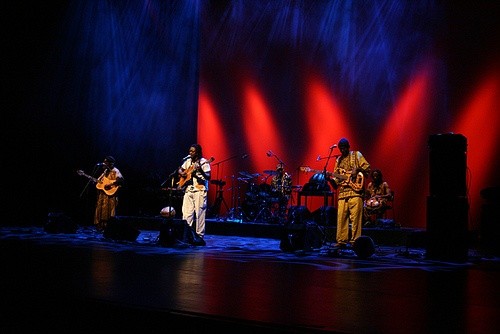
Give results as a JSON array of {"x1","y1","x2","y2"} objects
[
  {"x1": 93, "y1": 155, "x2": 125, "y2": 232},
  {"x1": 178, "y1": 144, "x2": 212, "y2": 239},
  {"x1": 270, "y1": 165, "x2": 294, "y2": 213},
  {"x1": 332, "y1": 138, "x2": 371, "y2": 248},
  {"x1": 368, "y1": 169, "x2": 391, "y2": 227}
]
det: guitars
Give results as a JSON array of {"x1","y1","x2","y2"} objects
[
  {"x1": 300, "y1": 166, "x2": 364, "y2": 192},
  {"x1": 179, "y1": 156, "x2": 214, "y2": 188},
  {"x1": 75, "y1": 170, "x2": 121, "y2": 197}
]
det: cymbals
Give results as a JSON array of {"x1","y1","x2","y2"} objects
[
  {"x1": 262, "y1": 170, "x2": 278, "y2": 175},
  {"x1": 237, "y1": 177, "x2": 250, "y2": 181},
  {"x1": 250, "y1": 173, "x2": 260, "y2": 178},
  {"x1": 240, "y1": 170, "x2": 254, "y2": 179}
]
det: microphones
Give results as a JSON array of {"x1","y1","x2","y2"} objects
[
  {"x1": 241, "y1": 155, "x2": 248, "y2": 159},
  {"x1": 97, "y1": 163, "x2": 105, "y2": 165},
  {"x1": 183, "y1": 155, "x2": 191, "y2": 160},
  {"x1": 331, "y1": 144, "x2": 338, "y2": 149},
  {"x1": 267, "y1": 152, "x2": 272, "y2": 157}
]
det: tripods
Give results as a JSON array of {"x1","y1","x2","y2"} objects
[{"x1": 252, "y1": 172, "x2": 276, "y2": 223}]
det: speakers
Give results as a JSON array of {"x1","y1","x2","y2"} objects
[
  {"x1": 279, "y1": 223, "x2": 324, "y2": 253},
  {"x1": 426, "y1": 134, "x2": 469, "y2": 262},
  {"x1": 103, "y1": 216, "x2": 193, "y2": 247}
]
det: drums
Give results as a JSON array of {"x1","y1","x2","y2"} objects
[
  {"x1": 271, "y1": 189, "x2": 281, "y2": 203},
  {"x1": 257, "y1": 183, "x2": 272, "y2": 197},
  {"x1": 240, "y1": 198, "x2": 262, "y2": 220},
  {"x1": 245, "y1": 184, "x2": 257, "y2": 197}
]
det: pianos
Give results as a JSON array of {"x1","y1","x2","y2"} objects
[{"x1": 160, "y1": 186, "x2": 186, "y2": 238}]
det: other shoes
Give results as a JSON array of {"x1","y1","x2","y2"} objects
[{"x1": 332, "y1": 243, "x2": 343, "y2": 247}]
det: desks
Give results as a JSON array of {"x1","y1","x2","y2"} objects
[{"x1": 297, "y1": 191, "x2": 334, "y2": 228}]
